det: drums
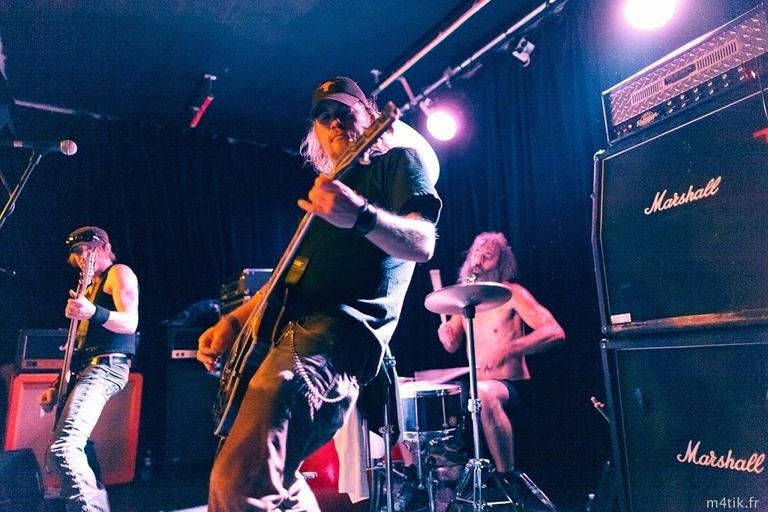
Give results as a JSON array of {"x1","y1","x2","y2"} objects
[{"x1": 399, "y1": 381, "x2": 464, "y2": 441}]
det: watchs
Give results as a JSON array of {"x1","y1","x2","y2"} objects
[{"x1": 352, "y1": 202, "x2": 377, "y2": 238}]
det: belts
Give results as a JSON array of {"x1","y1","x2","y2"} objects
[{"x1": 72, "y1": 355, "x2": 128, "y2": 373}]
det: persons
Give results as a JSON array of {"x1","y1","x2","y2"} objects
[
  {"x1": 38, "y1": 225, "x2": 140, "y2": 511},
  {"x1": 194, "y1": 76, "x2": 443, "y2": 512},
  {"x1": 434, "y1": 232, "x2": 567, "y2": 509}
]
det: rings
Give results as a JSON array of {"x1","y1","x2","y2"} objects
[{"x1": 68, "y1": 309, "x2": 73, "y2": 315}]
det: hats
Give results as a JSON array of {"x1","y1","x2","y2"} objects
[
  {"x1": 64, "y1": 226, "x2": 109, "y2": 252},
  {"x1": 308, "y1": 76, "x2": 367, "y2": 120}
]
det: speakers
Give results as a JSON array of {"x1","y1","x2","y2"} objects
[
  {"x1": 596, "y1": 323, "x2": 767, "y2": 511},
  {"x1": 589, "y1": 70, "x2": 768, "y2": 334}
]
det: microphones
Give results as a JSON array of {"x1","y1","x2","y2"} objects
[{"x1": 12, "y1": 137, "x2": 80, "y2": 158}]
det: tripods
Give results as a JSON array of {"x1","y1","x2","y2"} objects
[{"x1": 443, "y1": 338, "x2": 519, "y2": 512}]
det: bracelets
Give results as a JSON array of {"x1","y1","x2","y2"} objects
[
  {"x1": 227, "y1": 314, "x2": 242, "y2": 339},
  {"x1": 91, "y1": 305, "x2": 109, "y2": 325}
]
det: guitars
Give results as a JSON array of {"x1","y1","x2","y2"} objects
[
  {"x1": 213, "y1": 101, "x2": 400, "y2": 438},
  {"x1": 50, "y1": 251, "x2": 96, "y2": 442}
]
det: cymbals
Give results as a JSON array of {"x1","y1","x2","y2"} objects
[{"x1": 425, "y1": 282, "x2": 512, "y2": 315}]
